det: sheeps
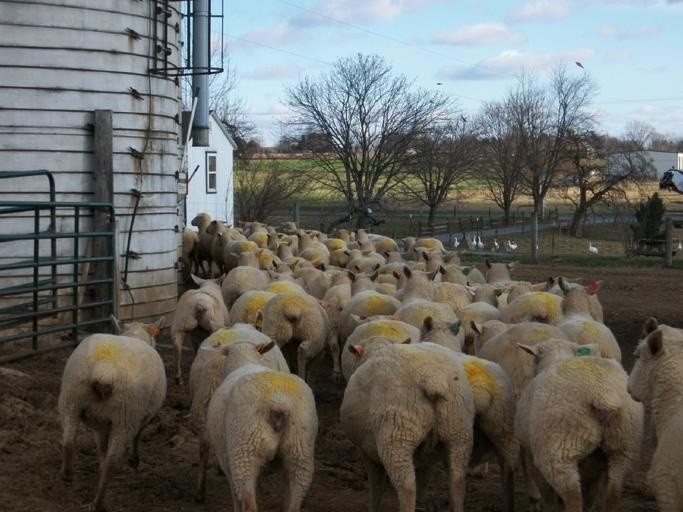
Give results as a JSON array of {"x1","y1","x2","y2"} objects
[
  {"x1": 170, "y1": 212, "x2": 682, "y2": 512},
  {"x1": 55, "y1": 313, "x2": 169, "y2": 511}
]
